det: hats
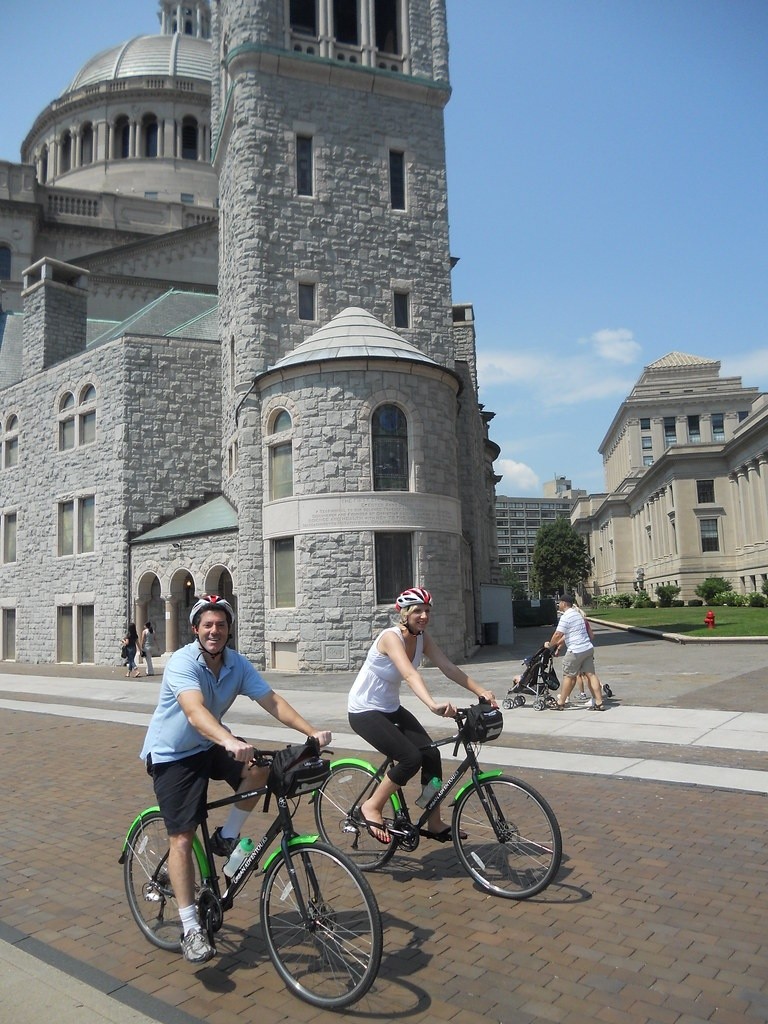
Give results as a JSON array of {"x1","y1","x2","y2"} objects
[{"x1": 557, "y1": 595, "x2": 574, "y2": 603}]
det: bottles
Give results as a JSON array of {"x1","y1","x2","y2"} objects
[
  {"x1": 223, "y1": 838, "x2": 254, "y2": 878},
  {"x1": 417, "y1": 777, "x2": 444, "y2": 809}
]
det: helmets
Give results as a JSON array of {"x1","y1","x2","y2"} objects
[
  {"x1": 396, "y1": 586, "x2": 433, "y2": 610},
  {"x1": 189, "y1": 595, "x2": 235, "y2": 627}
]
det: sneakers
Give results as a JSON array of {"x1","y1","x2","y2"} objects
[
  {"x1": 181, "y1": 927, "x2": 216, "y2": 966},
  {"x1": 574, "y1": 692, "x2": 587, "y2": 700},
  {"x1": 585, "y1": 697, "x2": 598, "y2": 705},
  {"x1": 208, "y1": 825, "x2": 259, "y2": 870},
  {"x1": 603, "y1": 684, "x2": 612, "y2": 697},
  {"x1": 557, "y1": 694, "x2": 570, "y2": 704}
]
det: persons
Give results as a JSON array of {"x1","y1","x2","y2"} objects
[
  {"x1": 122, "y1": 623, "x2": 143, "y2": 677},
  {"x1": 140, "y1": 595, "x2": 332, "y2": 962},
  {"x1": 347, "y1": 588, "x2": 497, "y2": 845},
  {"x1": 544, "y1": 595, "x2": 604, "y2": 711},
  {"x1": 514, "y1": 675, "x2": 523, "y2": 683},
  {"x1": 140, "y1": 621, "x2": 159, "y2": 676}
]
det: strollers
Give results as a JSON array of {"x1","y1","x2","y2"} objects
[{"x1": 499, "y1": 640, "x2": 562, "y2": 711}]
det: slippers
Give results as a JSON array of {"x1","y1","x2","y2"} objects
[
  {"x1": 125, "y1": 674, "x2": 130, "y2": 677},
  {"x1": 134, "y1": 672, "x2": 140, "y2": 678},
  {"x1": 427, "y1": 826, "x2": 468, "y2": 840},
  {"x1": 358, "y1": 805, "x2": 392, "y2": 845}
]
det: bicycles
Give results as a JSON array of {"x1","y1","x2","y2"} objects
[
  {"x1": 305, "y1": 694, "x2": 564, "y2": 899},
  {"x1": 116, "y1": 733, "x2": 386, "y2": 1013}
]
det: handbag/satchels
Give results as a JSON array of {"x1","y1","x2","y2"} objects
[
  {"x1": 463, "y1": 703, "x2": 503, "y2": 743},
  {"x1": 121, "y1": 645, "x2": 126, "y2": 658},
  {"x1": 267, "y1": 736, "x2": 331, "y2": 799},
  {"x1": 540, "y1": 668, "x2": 560, "y2": 690}
]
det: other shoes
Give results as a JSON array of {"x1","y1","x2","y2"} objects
[{"x1": 145, "y1": 673, "x2": 154, "y2": 676}]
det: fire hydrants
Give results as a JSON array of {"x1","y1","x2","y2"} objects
[{"x1": 704, "y1": 610, "x2": 715, "y2": 629}]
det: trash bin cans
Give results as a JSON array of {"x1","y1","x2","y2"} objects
[{"x1": 481, "y1": 622, "x2": 498, "y2": 645}]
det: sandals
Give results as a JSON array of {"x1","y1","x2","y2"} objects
[
  {"x1": 549, "y1": 701, "x2": 564, "y2": 710},
  {"x1": 587, "y1": 702, "x2": 605, "y2": 711}
]
